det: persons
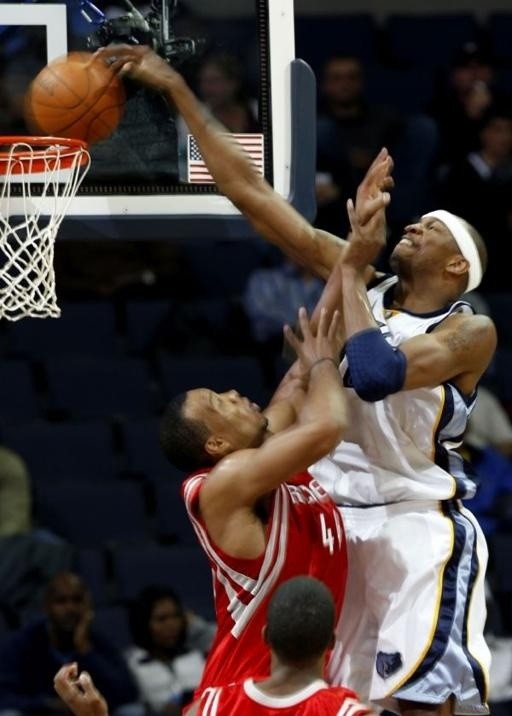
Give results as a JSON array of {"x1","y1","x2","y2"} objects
[
  {"x1": 1, "y1": 446, "x2": 32, "y2": 572},
  {"x1": 53, "y1": 661, "x2": 109, "y2": 716},
  {"x1": 158, "y1": 147, "x2": 394, "y2": 715},
  {"x1": 120, "y1": 584, "x2": 206, "y2": 716},
  {"x1": 2, "y1": 570, "x2": 140, "y2": 716},
  {"x1": 88, "y1": 41, "x2": 495, "y2": 715},
  {"x1": 173, "y1": 44, "x2": 511, "y2": 448},
  {"x1": 483, "y1": 575, "x2": 512, "y2": 703}
]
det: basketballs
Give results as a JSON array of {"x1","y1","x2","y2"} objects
[{"x1": 30, "y1": 51, "x2": 124, "y2": 145}]
